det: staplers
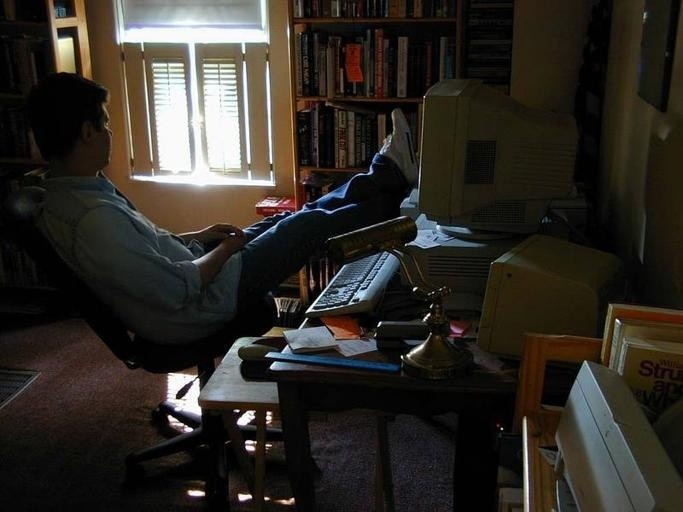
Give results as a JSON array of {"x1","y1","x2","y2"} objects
[{"x1": 373, "y1": 321, "x2": 430, "y2": 349}]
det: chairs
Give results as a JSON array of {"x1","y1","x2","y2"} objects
[{"x1": 1, "y1": 161, "x2": 283, "y2": 512}]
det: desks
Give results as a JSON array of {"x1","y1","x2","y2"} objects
[{"x1": 264, "y1": 285, "x2": 520, "y2": 512}]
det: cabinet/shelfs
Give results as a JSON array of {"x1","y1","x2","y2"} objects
[
  {"x1": 285, "y1": 0, "x2": 514, "y2": 215},
  {"x1": 0, "y1": 0, "x2": 95, "y2": 160},
  {"x1": 197, "y1": 336, "x2": 280, "y2": 512}
]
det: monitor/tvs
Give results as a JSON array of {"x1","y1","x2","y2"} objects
[
  {"x1": 477, "y1": 233, "x2": 630, "y2": 360},
  {"x1": 417, "y1": 79, "x2": 579, "y2": 238}
]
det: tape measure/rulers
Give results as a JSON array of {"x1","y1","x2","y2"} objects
[{"x1": 265, "y1": 352, "x2": 399, "y2": 373}]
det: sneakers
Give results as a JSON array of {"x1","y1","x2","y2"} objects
[{"x1": 377, "y1": 106, "x2": 418, "y2": 185}]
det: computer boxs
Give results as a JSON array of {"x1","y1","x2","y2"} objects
[{"x1": 397, "y1": 214, "x2": 539, "y2": 312}]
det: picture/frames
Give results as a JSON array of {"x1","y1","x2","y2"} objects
[{"x1": 638, "y1": 0, "x2": 682, "y2": 113}]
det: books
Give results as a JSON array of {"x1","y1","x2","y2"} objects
[
  {"x1": 598, "y1": 299, "x2": 683, "y2": 422},
  {"x1": 289, "y1": 2, "x2": 512, "y2": 318}
]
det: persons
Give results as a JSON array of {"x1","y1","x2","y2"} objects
[{"x1": 20, "y1": 73, "x2": 419, "y2": 351}]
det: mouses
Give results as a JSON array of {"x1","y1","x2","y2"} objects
[{"x1": 238, "y1": 344, "x2": 281, "y2": 363}]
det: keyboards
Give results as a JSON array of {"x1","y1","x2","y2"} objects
[{"x1": 303, "y1": 247, "x2": 400, "y2": 320}]
